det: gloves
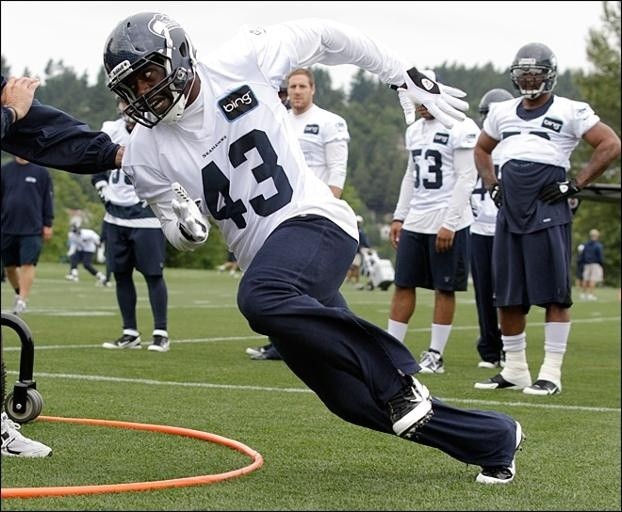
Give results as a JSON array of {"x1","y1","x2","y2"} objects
[
  {"x1": 171, "y1": 182, "x2": 206, "y2": 242},
  {"x1": 489, "y1": 181, "x2": 502, "y2": 208},
  {"x1": 97, "y1": 187, "x2": 108, "y2": 205},
  {"x1": 538, "y1": 177, "x2": 581, "y2": 205},
  {"x1": 388, "y1": 66, "x2": 469, "y2": 129}
]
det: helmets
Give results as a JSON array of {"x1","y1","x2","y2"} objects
[
  {"x1": 279, "y1": 80, "x2": 291, "y2": 109},
  {"x1": 478, "y1": 88, "x2": 516, "y2": 113},
  {"x1": 69, "y1": 216, "x2": 83, "y2": 231},
  {"x1": 510, "y1": 43, "x2": 558, "y2": 100},
  {"x1": 103, "y1": 12, "x2": 197, "y2": 129}
]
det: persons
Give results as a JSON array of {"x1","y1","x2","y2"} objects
[
  {"x1": 278, "y1": 85, "x2": 291, "y2": 109},
  {"x1": 63, "y1": 217, "x2": 115, "y2": 286},
  {"x1": 214, "y1": 244, "x2": 240, "y2": 277},
  {"x1": 0, "y1": 66, "x2": 125, "y2": 459},
  {"x1": 104, "y1": 12, "x2": 526, "y2": 484},
  {"x1": 386, "y1": 68, "x2": 480, "y2": 375},
  {"x1": 1, "y1": 155, "x2": 55, "y2": 315},
  {"x1": 92, "y1": 96, "x2": 168, "y2": 353},
  {"x1": 473, "y1": 42, "x2": 622, "y2": 396},
  {"x1": 247, "y1": 69, "x2": 351, "y2": 360},
  {"x1": 344, "y1": 214, "x2": 370, "y2": 290}
]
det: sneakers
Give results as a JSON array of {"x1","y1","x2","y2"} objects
[
  {"x1": 64, "y1": 272, "x2": 112, "y2": 288},
  {"x1": 102, "y1": 329, "x2": 142, "y2": 350},
  {"x1": 474, "y1": 419, "x2": 526, "y2": 484},
  {"x1": 12, "y1": 295, "x2": 28, "y2": 315},
  {"x1": 383, "y1": 374, "x2": 435, "y2": 440},
  {"x1": 148, "y1": 329, "x2": 170, "y2": 353},
  {"x1": 0, "y1": 410, "x2": 53, "y2": 458},
  {"x1": 245, "y1": 345, "x2": 283, "y2": 360},
  {"x1": 418, "y1": 350, "x2": 558, "y2": 396}
]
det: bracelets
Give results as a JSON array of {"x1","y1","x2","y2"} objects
[{"x1": 9, "y1": 106, "x2": 19, "y2": 124}]
